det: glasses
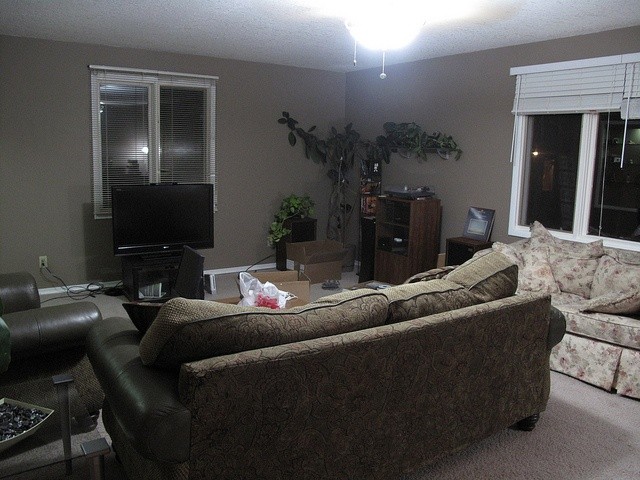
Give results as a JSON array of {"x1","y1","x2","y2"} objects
[{"x1": 346, "y1": 6, "x2": 427, "y2": 80}]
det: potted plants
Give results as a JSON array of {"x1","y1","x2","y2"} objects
[{"x1": 462, "y1": 206, "x2": 496, "y2": 242}]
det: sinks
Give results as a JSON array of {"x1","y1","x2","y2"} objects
[
  {"x1": 1, "y1": 272, "x2": 104, "y2": 443},
  {"x1": 86, "y1": 248, "x2": 566, "y2": 478},
  {"x1": 495, "y1": 238, "x2": 639, "y2": 401}
]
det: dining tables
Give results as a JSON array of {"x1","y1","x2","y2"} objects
[
  {"x1": 265, "y1": 193, "x2": 315, "y2": 248},
  {"x1": 355, "y1": 121, "x2": 462, "y2": 285},
  {"x1": 278, "y1": 111, "x2": 361, "y2": 272}
]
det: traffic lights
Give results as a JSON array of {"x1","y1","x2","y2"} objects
[
  {"x1": 492, "y1": 239, "x2": 561, "y2": 295},
  {"x1": 531, "y1": 220, "x2": 603, "y2": 299},
  {"x1": 122, "y1": 303, "x2": 162, "y2": 337},
  {"x1": 579, "y1": 248, "x2": 639, "y2": 313}
]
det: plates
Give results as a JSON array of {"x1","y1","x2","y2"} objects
[{"x1": 374, "y1": 197, "x2": 441, "y2": 285}]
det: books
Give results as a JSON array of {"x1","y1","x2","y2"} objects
[
  {"x1": 242, "y1": 271, "x2": 311, "y2": 308},
  {"x1": 286, "y1": 239, "x2": 342, "y2": 283}
]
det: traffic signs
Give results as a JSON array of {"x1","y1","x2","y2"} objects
[{"x1": 39, "y1": 255, "x2": 47, "y2": 269}]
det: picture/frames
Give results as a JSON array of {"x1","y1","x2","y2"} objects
[{"x1": 123, "y1": 251, "x2": 183, "y2": 302}]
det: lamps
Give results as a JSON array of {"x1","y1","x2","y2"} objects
[{"x1": 144, "y1": 245, "x2": 204, "y2": 302}]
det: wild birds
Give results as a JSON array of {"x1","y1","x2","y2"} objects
[{"x1": 122, "y1": 245, "x2": 205, "y2": 332}]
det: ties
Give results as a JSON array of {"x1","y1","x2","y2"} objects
[{"x1": 111, "y1": 183, "x2": 214, "y2": 256}]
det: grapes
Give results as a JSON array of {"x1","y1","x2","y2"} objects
[
  {"x1": 276, "y1": 217, "x2": 317, "y2": 270},
  {"x1": 445, "y1": 237, "x2": 495, "y2": 267}
]
